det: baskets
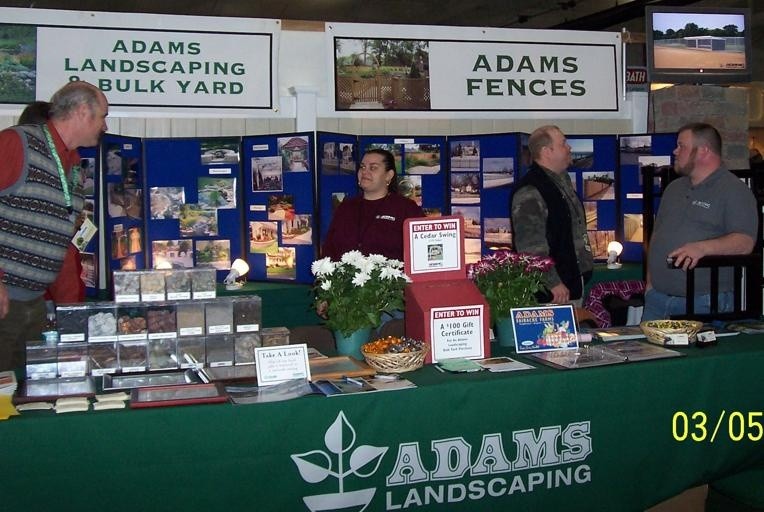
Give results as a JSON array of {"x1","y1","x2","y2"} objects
[
  {"x1": 640, "y1": 320, "x2": 703, "y2": 346},
  {"x1": 360, "y1": 342, "x2": 431, "y2": 374}
]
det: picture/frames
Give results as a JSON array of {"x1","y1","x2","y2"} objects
[
  {"x1": 129, "y1": 379, "x2": 228, "y2": 408},
  {"x1": 254, "y1": 342, "x2": 377, "y2": 387}
]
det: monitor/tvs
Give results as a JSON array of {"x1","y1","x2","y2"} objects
[{"x1": 645, "y1": 5, "x2": 753, "y2": 84}]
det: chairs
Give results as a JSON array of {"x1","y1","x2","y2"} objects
[{"x1": 593, "y1": 250, "x2": 761, "y2": 325}]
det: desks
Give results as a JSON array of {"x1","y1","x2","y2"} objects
[{"x1": 0, "y1": 314, "x2": 764, "y2": 512}]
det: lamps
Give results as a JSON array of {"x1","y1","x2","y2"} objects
[
  {"x1": 223, "y1": 259, "x2": 251, "y2": 290},
  {"x1": 605, "y1": 241, "x2": 624, "y2": 269}
]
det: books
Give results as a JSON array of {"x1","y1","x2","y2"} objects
[{"x1": 310, "y1": 373, "x2": 418, "y2": 398}]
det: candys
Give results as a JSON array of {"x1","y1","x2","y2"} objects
[{"x1": 363, "y1": 335, "x2": 426, "y2": 354}]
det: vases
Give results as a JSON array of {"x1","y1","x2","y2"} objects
[
  {"x1": 331, "y1": 325, "x2": 374, "y2": 363},
  {"x1": 494, "y1": 315, "x2": 516, "y2": 348}
]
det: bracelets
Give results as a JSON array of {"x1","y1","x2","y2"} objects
[{"x1": 686, "y1": 256, "x2": 692, "y2": 260}]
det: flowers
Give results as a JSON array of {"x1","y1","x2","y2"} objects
[
  {"x1": 464, "y1": 250, "x2": 558, "y2": 329},
  {"x1": 305, "y1": 250, "x2": 415, "y2": 341}
]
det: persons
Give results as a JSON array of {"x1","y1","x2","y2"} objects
[
  {"x1": 472, "y1": 147, "x2": 478, "y2": 157},
  {"x1": 639, "y1": 122, "x2": 759, "y2": 325},
  {"x1": 314, "y1": 149, "x2": 427, "y2": 361},
  {"x1": 510, "y1": 123, "x2": 593, "y2": 308},
  {"x1": 457, "y1": 142, "x2": 463, "y2": 157},
  {"x1": 80, "y1": 258, "x2": 87, "y2": 280},
  {"x1": 270, "y1": 201, "x2": 305, "y2": 234},
  {"x1": 0, "y1": 82, "x2": 108, "y2": 373},
  {"x1": 327, "y1": 144, "x2": 334, "y2": 160},
  {"x1": 19, "y1": 99, "x2": 85, "y2": 308},
  {"x1": 109, "y1": 151, "x2": 121, "y2": 175}
]
